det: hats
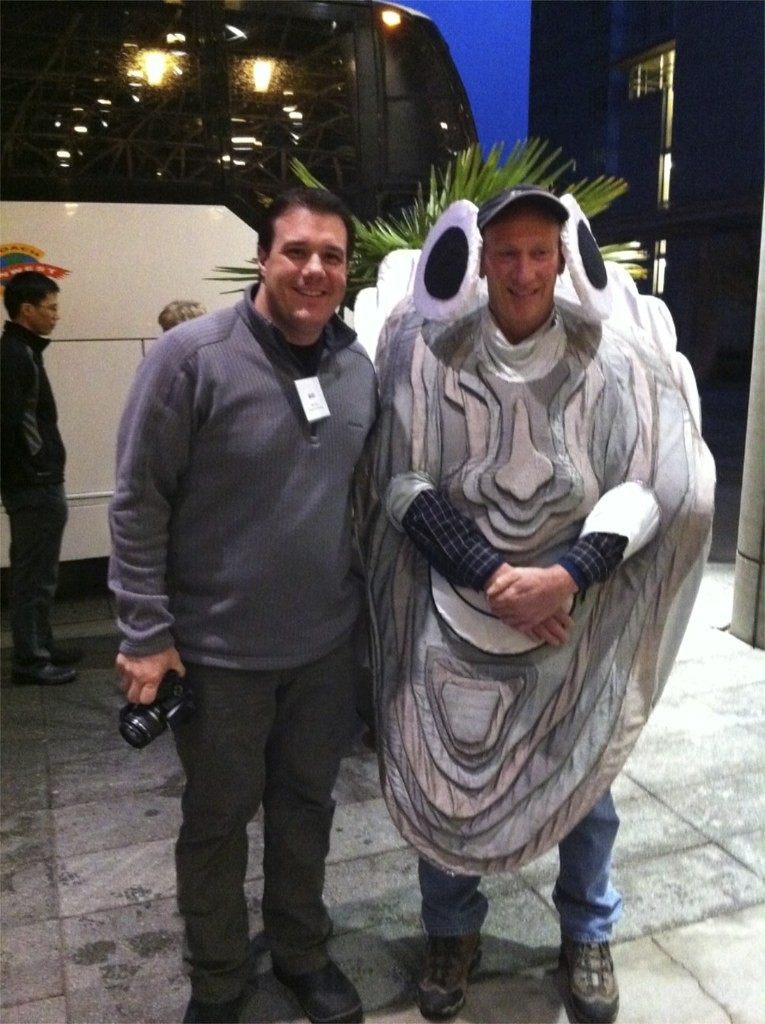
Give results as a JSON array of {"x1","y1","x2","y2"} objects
[{"x1": 477, "y1": 186, "x2": 570, "y2": 233}]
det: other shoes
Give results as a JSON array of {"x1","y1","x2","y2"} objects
[
  {"x1": 53, "y1": 650, "x2": 85, "y2": 665},
  {"x1": 13, "y1": 661, "x2": 77, "y2": 683},
  {"x1": 274, "y1": 945, "x2": 363, "y2": 1024},
  {"x1": 182, "y1": 970, "x2": 258, "y2": 1024}
]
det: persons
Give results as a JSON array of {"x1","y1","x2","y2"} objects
[
  {"x1": 105, "y1": 184, "x2": 378, "y2": 1024},
  {"x1": 376, "y1": 183, "x2": 663, "y2": 1024},
  {"x1": 0, "y1": 270, "x2": 88, "y2": 688}
]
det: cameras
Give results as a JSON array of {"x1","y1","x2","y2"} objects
[{"x1": 120, "y1": 669, "x2": 197, "y2": 749}]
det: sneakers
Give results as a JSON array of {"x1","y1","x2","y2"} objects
[
  {"x1": 560, "y1": 939, "x2": 616, "y2": 1023},
  {"x1": 416, "y1": 932, "x2": 482, "y2": 1018}
]
